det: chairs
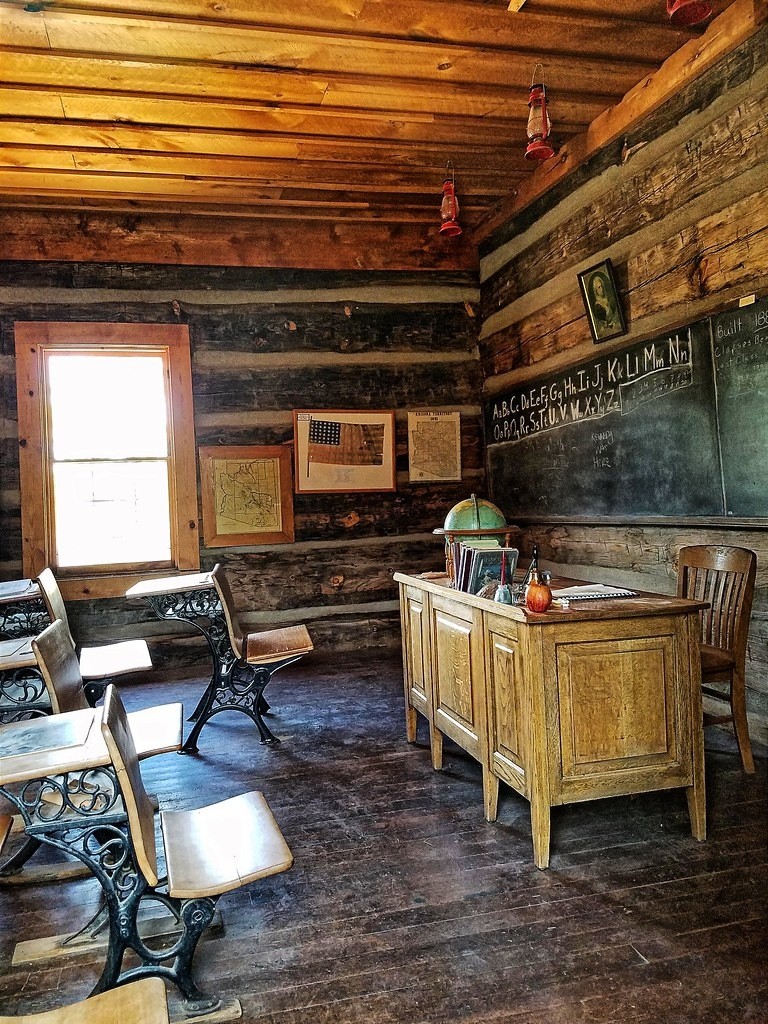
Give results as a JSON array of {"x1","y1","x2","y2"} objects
[
  {"x1": 676, "y1": 544, "x2": 756, "y2": 773},
  {"x1": 212, "y1": 562, "x2": 314, "y2": 746},
  {"x1": 30, "y1": 619, "x2": 183, "y2": 880},
  {"x1": 102, "y1": 682, "x2": 294, "y2": 1024},
  {"x1": 0, "y1": 976, "x2": 170, "y2": 1024},
  {"x1": 37, "y1": 567, "x2": 153, "y2": 707}
]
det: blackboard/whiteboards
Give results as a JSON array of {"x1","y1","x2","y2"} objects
[{"x1": 481, "y1": 287, "x2": 768, "y2": 528}]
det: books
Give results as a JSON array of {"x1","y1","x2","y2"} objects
[
  {"x1": 551, "y1": 583, "x2": 640, "y2": 601},
  {"x1": 450, "y1": 540, "x2": 519, "y2": 594}
]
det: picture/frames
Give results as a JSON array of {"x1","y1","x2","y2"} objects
[
  {"x1": 294, "y1": 409, "x2": 397, "y2": 494},
  {"x1": 576, "y1": 258, "x2": 628, "y2": 345}
]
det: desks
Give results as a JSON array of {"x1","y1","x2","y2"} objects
[
  {"x1": 392, "y1": 571, "x2": 712, "y2": 872},
  {"x1": 0, "y1": 633, "x2": 43, "y2": 722},
  {"x1": 0, "y1": 704, "x2": 112, "y2": 1000},
  {"x1": 0, "y1": 579, "x2": 52, "y2": 641},
  {"x1": 125, "y1": 572, "x2": 212, "y2": 756}
]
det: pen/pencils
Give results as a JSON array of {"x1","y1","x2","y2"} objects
[
  {"x1": 516, "y1": 559, "x2": 535, "y2": 601},
  {"x1": 501, "y1": 550, "x2": 505, "y2": 585}
]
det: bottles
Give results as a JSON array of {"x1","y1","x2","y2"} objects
[{"x1": 494, "y1": 585, "x2": 511, "y2": 603}]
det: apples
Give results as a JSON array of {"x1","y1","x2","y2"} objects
[{"x1": 526, "y1": 583, "x2": 552, "y2": 611}]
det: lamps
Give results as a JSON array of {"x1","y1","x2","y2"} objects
[
  {"x1": 438, "y1": 160, "x2": 462, "y2": 236},
  {"x1": 524, "y1": 64, "x2": 555, "y2": 162}
]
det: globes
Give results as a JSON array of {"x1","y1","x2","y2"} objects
[{"x1": 432, "y1": 493, "x2": 522, "y2": 578}]
